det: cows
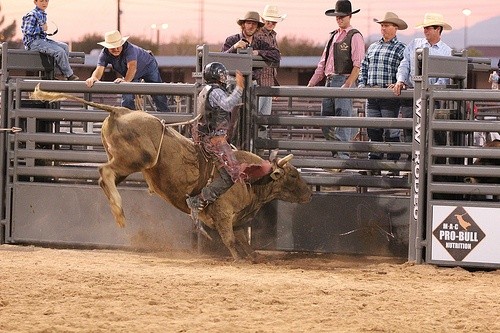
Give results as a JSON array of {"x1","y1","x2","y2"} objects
[{"x1": 30, "y1": 82, "x2": 314, "y2": 265}]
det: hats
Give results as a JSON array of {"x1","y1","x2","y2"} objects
[
  {"x1": 260, "y1": 4, "x2": 288, "y2": 23},
  {"x1": 373, "y1": 11, "x2": 408, "y2": 31},
  {"x1": 414, "y1": 14, "x2": 453, "y2": 31},
  {"x1": 324, "y1": 0, "x2": 361, "y2": 17},
  {"x1": 96, "y1": 30, "x2": 129, "y2": 49},
  {"x1": 236, "y1": 11, "x2": 265, "y2": 32}
]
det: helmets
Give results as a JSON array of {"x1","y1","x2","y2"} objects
[{"x1": 202, "y1": 61, "x2": 231, "y2": 90}]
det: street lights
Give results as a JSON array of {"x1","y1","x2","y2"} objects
[
  {"x1": 462, "y1": 8, "x2": 472, "y2": 49},
  {"x1": 150, "y1": 23, "x2": 168, "y2": 56}
]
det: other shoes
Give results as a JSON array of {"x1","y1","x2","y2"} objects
[
  {"x1": 67, "y1": 74, "x2": 79, "y2": 81},
  {"x1": 185, "y1": 194, "x2": 209, "y2": 221}
]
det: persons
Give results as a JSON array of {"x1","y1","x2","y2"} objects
[
  {"x1": 390, "y1": 12, "x2": 453, "y2": 171},
  {"x1": 86, "y1": 30, "x2": 170, "y2": 112},
  {"x1": 184, "y1": 61, "x2": 246, "y2": 223},
  {"x1": 21, "y1": 0, "x2": 82, "y2": 82},
  {"x1": 307, "y1": 0, "x2": 366, "y2": 170},
  {"x1": 358, "y1": 11, "x2": 409, "y2": 176},
  {"x1": 255, "y1": 3, "x2": 288, "y2": 138},
  {"x1": 222, "y1": 12, "x2": 281, "y2": 148}
]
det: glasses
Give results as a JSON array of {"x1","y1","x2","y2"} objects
[{"x1": 45, "y1": 22, "x2": 59, "y2": 36}]
